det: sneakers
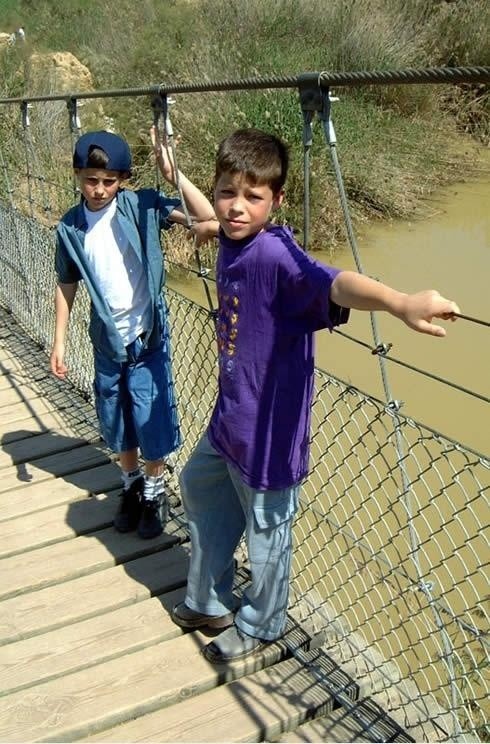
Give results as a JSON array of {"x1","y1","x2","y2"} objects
[
  {"x1": 171, "y1": 600, "x2": 235, "y2": 631},
  {"x1": 138, "y1": 492, "x2": 168, "y2": 539},
  {"x1": 114, "y1": 477, "x2": 145, "y2": 533},
  {"x1": 202, "y1": 626, "x2": 263, "y2": 665}
]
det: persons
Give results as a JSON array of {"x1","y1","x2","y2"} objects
[
  {"x1": 49, "y1": 124, "x2": 215, "y2": 541},
  {"x1": 170, "y1": 128, "x2": 459, "y2": 665}
]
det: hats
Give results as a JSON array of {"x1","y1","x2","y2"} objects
[{"x1": 73, "y1": 131, "x2": 133, "y2": 179}]
